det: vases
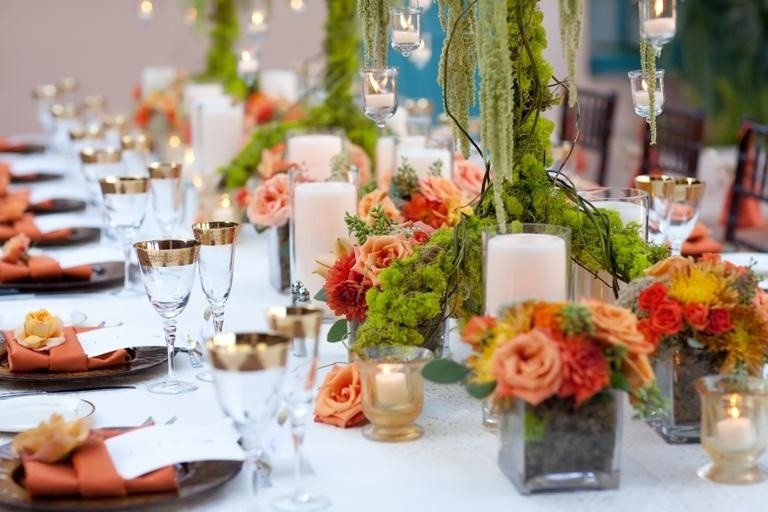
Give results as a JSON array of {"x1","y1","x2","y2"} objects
[
  {"x1": 630, "y1": 329, "x2": 748, "y2": 445},
  {"x1": 491, "y1": 378, "x2": 628, "y2": 497}
]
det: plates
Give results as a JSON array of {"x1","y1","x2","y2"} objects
[
  {"x1": 1, "y1": 393, "x2": 95, "y2": 435},
  {"x1": 719, "y1": 252, "x2": 768, "y2": 292},
  {"x1": 0, "y1": 138, "x2": 88, "y2": 342}
]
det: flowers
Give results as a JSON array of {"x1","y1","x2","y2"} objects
[
  {"x1": 412, "y1": 292, "x2": 670, "y2": 439},
  {"x1": 1, "y1": 232, "x2": 31, "y2": 264},
  {"x1": 15, "y1": 306, "x2": 68, "y2": 354},
  {"x1": 13, "y1": 410, "x2": 92, "y2": 466},
  {"x1": 133, "y1": 80, "x2": 301, "y2": 197},
  {"x1": 607, "y1": 245, "x2": 766, "y2": 383},
  {"x1": 243, "y1": 94, "x2": 490, "y2": 325}
]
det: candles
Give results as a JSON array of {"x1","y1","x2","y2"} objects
[
  {"x1": 711, "y1": 412, "x2": 757, "y2": 451},
  {"x1": 387, "y1": 24, "x2": 422, "y2": 48},
  {"x1": 481, "y1": 229, "x2": 567, "y2": 326},
  {"x1": 632, "y1": 88, "x2": 661, "y2": 112},
  {"x1": 641, "y1": 13, "x2": 676, "y2": 39}
]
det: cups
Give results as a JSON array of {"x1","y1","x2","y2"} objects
[
  {"x1": 633, "y1": 172, "x2": 705, "y2": 258},
  {"x1": 351, "y1": 342, "x2": 437, "y2": 446},
  {"x1": 190, "y1": 69, "x2": 461, "y2": 333},
  {"x1": 687, "y1": 370, "x2": 766, "y2": 488},
  {"x1": 478, "y1": 219, "x2": 574, "y2": 437},
  {"x1": 578, "y1": 184, "x2": 651, "y2": 242}
]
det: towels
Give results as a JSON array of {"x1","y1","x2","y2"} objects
[
  {"x1": 0, "y1": 208, "x2": 75, "y2": 245},
  {"x1": 0, "y1": 137, "x2": 56, "y2": 216},
  {"x1": 0, "y1": 322, "x2": 140, "y2": 373},
  {"x1": 0, "y1": 254, "x2": 94, "y2": 287},
  {"x1": 10, "y1": 414, "x2": 182, "y2": 500}
]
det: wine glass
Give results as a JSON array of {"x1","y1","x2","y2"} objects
[
  {"x1": 191, "y1": 221, "x2": 241, "y2": 337},
  {"x1": 202, "y1": 305, "x2": 323, "y2": 511},
  {"x1": 33, "y1": 75, "x2": 190, "y2": 138},
  {"x1": 132, "y1": 239, "x2": 200, "y2": 394},
  {"x1": 35, "y1": 75, "x2": 184, "y2": 299}
]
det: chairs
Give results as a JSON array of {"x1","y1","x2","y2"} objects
[
  {"x1": 556, "y1": 85, "x2": 618, "y2": 187},
  {"x1": 723, "y1": 121, "x2": 767, "y2": 253},
  {"x1": 619, "y1": 103, "x2": 707, "y2": 220}
]
